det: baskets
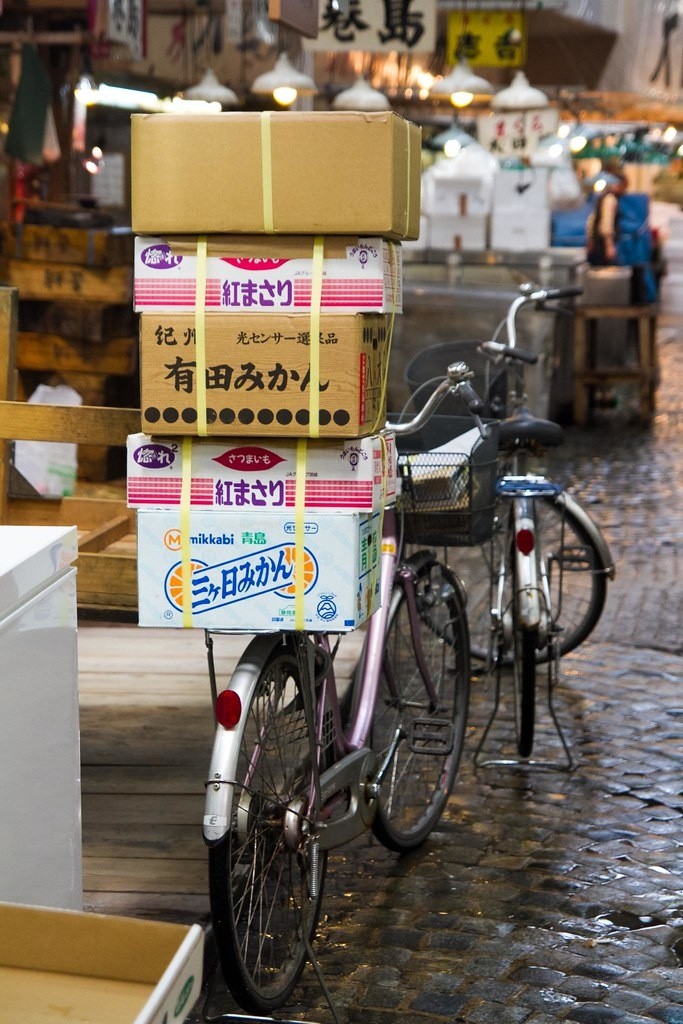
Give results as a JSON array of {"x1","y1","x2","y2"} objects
[
  {"x1": 405, "y1": 344, "x2": 509, "y2": 417},
  {"x1": 384, "y1": 411, "x2": 502, "y2": 547}
]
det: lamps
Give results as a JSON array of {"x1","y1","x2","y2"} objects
[
  {"x1": 432, "y1": 62, "x2": 495, "y2": 107},
  {"x1": 250, "y1": 52, "x2": 318, "y2": 106},
  {"x1": 336, "y1": 71, "x2": 392, "y2": 112},
  {"x1": 491, "y1": 71, "x2": 548, "y2": 110},
  {"x1": 188, "y1": 70, "x2": 239, "y2": 103}
]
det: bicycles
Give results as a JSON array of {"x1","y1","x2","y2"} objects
[
  {"x1": 401, "y1": 280, "x2": 619, "y2": 773},
  {"x1": 194, "y1": 358, "x2": 495, "y2": 1024}
]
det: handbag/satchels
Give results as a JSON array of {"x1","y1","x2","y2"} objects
[{"x1": 16, "y1": 385, "x2": 84, "y2": 500}]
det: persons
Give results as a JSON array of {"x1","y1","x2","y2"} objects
[{"x1": 587, "y1": 172, "x2": 628, "y2": 266}]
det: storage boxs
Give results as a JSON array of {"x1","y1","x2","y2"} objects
[
  {"x1": 125, "y1": 110, "x2": 551, "y2": 633},
  {"x1": 1, "y1": 897, "x2": 206, "y2": 1023}
]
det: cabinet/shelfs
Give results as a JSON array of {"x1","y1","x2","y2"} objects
[{"x1": 573, "y1": 303, "x2": 658, "y2": 425}]
